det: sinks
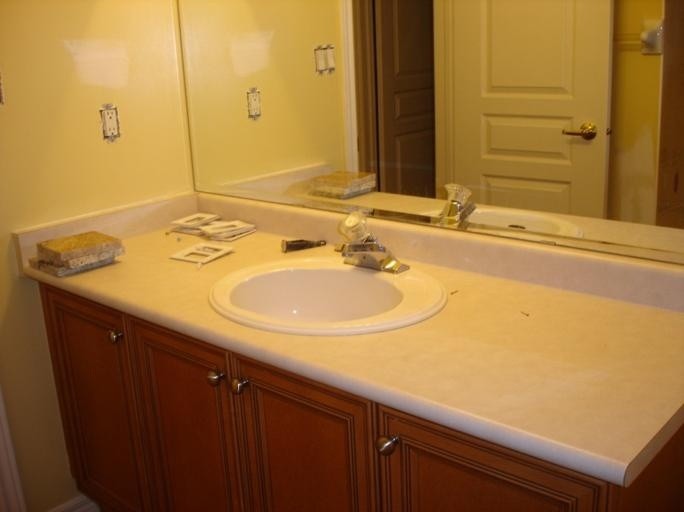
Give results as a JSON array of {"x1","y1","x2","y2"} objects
[
  {"x1": 415, "y1": 207, "x2": 582, "y2": 238},
  {"x1": 209, "y1": 257, "x2": 447, "y2": 336}
]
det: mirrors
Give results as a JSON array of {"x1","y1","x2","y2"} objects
[{"x1": 177, "y1": 0, "x2": 684, "y2": 267}]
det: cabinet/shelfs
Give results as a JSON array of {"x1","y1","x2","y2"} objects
[{"x1": 38, "y1": 281, "x2": 673, "y2": 512}]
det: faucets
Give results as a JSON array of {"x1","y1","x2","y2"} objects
[
  {"x1": 338, "y1": 210, "x2": 409, "y2": 274},
  {"x1": 438, "y1": 183, "x2": 476, "y2": 225}
]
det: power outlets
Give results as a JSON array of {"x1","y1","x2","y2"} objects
[
  {"x1": 100, "y1": 107, "x2": 120, "y2": 139},
  {"x1": 246, "y1": 90, "x2": 261, "y2": 117}
]
